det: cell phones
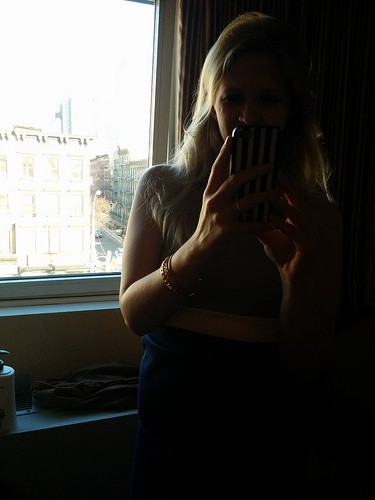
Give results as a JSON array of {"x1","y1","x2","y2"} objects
[{"x1": 228, "y1": 123, "x2": 280, "y2": 226}]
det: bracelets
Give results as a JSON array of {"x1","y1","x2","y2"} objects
[{"x1": 161, "y1": 256, "x2": 202, "y2": 298}]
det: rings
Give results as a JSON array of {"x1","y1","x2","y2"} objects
[{"x1": 234, "y1": 199, "x2": 244, "y2": 217}]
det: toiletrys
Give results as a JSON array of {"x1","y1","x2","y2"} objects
[{"x1": 0, "y1": 349, "x2": 15, "y2": 434}]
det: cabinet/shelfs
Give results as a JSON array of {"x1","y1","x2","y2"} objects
[{"x1": 2, "y1": 402, "x2": 138, "y2": 500}]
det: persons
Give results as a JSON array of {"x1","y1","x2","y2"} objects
[{"x1": 118, "y1": 10, "x2": 341, "y2": 500}]
